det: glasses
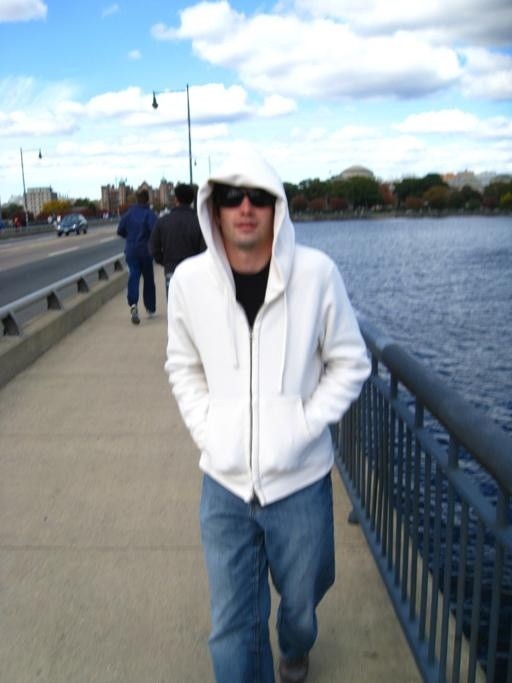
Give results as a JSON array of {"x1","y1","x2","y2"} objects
[{"x1": 214, "y1": 188, "x2": 274, "y2": 207}]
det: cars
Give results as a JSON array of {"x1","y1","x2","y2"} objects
[{"x1": 56, "y1": 213, "x2": 88, "y2": 237}]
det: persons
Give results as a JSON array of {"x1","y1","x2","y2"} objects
[
  {"x1": 116, "y1": 187, "x2": 158, "y2": 324},
  {"x1": 148, "y1": 183, "x2": 207, "y2": 304},
  {"x1": 163, "y1": 157, "x2": 373, "y2": 683}
]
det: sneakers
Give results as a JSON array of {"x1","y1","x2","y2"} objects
[
  {"x1": 131, "y1": 304, "x2": 140, "y2": 324},
  {"x1": 278, "y1": 656, "x2": 309, "y2": 681}
]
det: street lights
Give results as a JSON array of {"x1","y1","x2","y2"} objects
[
  {"x1": 194, "y1": 155, "x2": 211, "y2": 178},
  {"x1": 21, "y1": 148, "x2": 42, "y2": 232},
  {"x1": 152, "y1": 89, "x2": 194, "y2": 211},
  {"x1": 115, "y1": 175, "x2": 127, "y2": 219}
]
